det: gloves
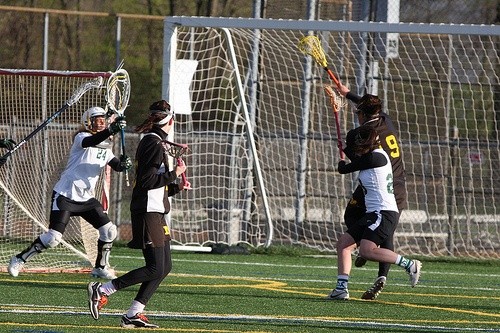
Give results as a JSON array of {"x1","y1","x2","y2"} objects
[
  {"x1": 108, "y1": 116, "x2": 127, "y2": 136},
  {"x1": 119, "y1": 155, "x2": 134, "y2": 171}
]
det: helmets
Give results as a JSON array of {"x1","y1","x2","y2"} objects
[{"x1": 82, "y1": 106, "x2": 106, "y2": 135}]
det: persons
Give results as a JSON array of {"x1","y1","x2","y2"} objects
[
  {"x1": 337, "y1": 82, "x2": 404, "y2": 300},
  {"x1": 87, "y1": 101, "x2": 191, "y2": 329},
  {"x1": 8, "y1": 107, "x2": 132, "y2": 279},
  {"x1": 322, "y1": 126, "x2": 422, "y2": 300},
  {"x1": 0, "y1": 139, "x2": 16, "y2": 168}
]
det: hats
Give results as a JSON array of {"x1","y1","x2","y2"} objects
[{"x1": 358, "y1": 94, "x2": 384, "y2": 117}]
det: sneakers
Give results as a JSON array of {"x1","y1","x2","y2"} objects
[
  {"x1": 7, "y1": 255, "x2": 25, "y2": 276},
  {"x1": 90, "y1": 266, "x2": 117, "y2": 280},
  {"x1": 119, "y1": 311, "x2": 160, "y2": 329},
  {"x1": 354, "y1": 254, "x2": 367, "y2": 267},
  {"x1": 322, "y1": 289, "x2": 349, "y2": 301},
  {"x1": 360, "y1": 277, "x2": 386, "y2": 301},
  {"x1": 87, "y1": 282, "x2": 108, "y2": 320},
  {"x1": 407, "y1": 259, "x2": 423, "y2": 288}
]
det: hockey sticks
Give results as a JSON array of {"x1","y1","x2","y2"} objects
[
  {"x1": 324, "y1": 86, "x2": 345, "y2": 159},
  {"x1": 297, "y1": 36, "x2": 341, "y2": 87},
  {"x1": 106, "y1": 68, "x2": 132, "y2": 188},
  {"x1": 162, "y1": 138, "x2": 190, "y2": 189},
  {"x1": 2, "y1": 75, "x2": 103, "y2": 160}
]
